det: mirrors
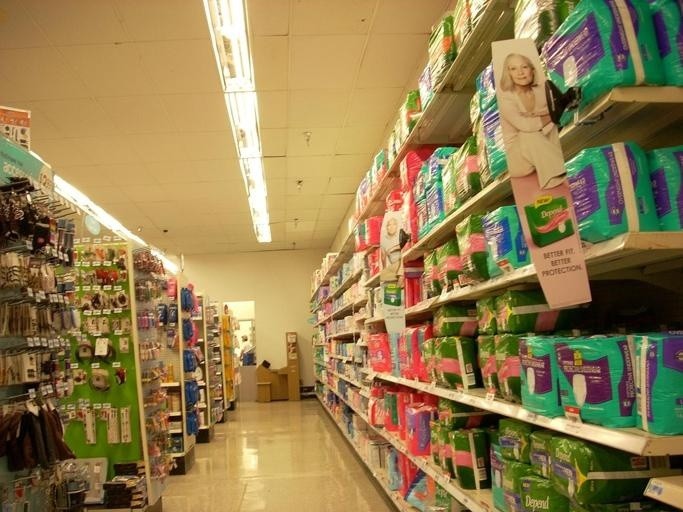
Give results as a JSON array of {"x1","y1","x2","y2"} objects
[{"x1": 221, "y1": 301, "x2": 257, "y2": 367}]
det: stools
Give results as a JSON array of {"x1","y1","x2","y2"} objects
[{"x1": 256, "y1": 381, "x2": 273, "y2": 402}]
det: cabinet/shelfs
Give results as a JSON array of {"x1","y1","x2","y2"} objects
[
  {"x1": 222, "y1": 301, "x2": 235, "y2": 411},
  {"x1": 159, "y1": 271, "x2": 196, "y2": 477},
  {"x1": 364, "y1": 1, "x2": 680, "y2": 512},
  {"x1": 192, "y1": 295, "x2": 215, "y2": 443},
  {"x1": 57, "y1": 241, "x2": 164, "y2": 512},
  {"x1": 313, "y1": 212, "x2": 362, "y2": 464},
  {"x1": 204, "y1": 301, "x2": 228, "y2": 424},
  {"x1": 1, "y1": 133, "x2": 82, "y2": 512}
]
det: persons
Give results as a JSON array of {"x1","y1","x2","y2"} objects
[
  {"x1": 499, "y1": 53, "x2": 567, "y2": 192},
  {"x1": 239, "y1": 336, "x2": 252, "y2": 366}
]
